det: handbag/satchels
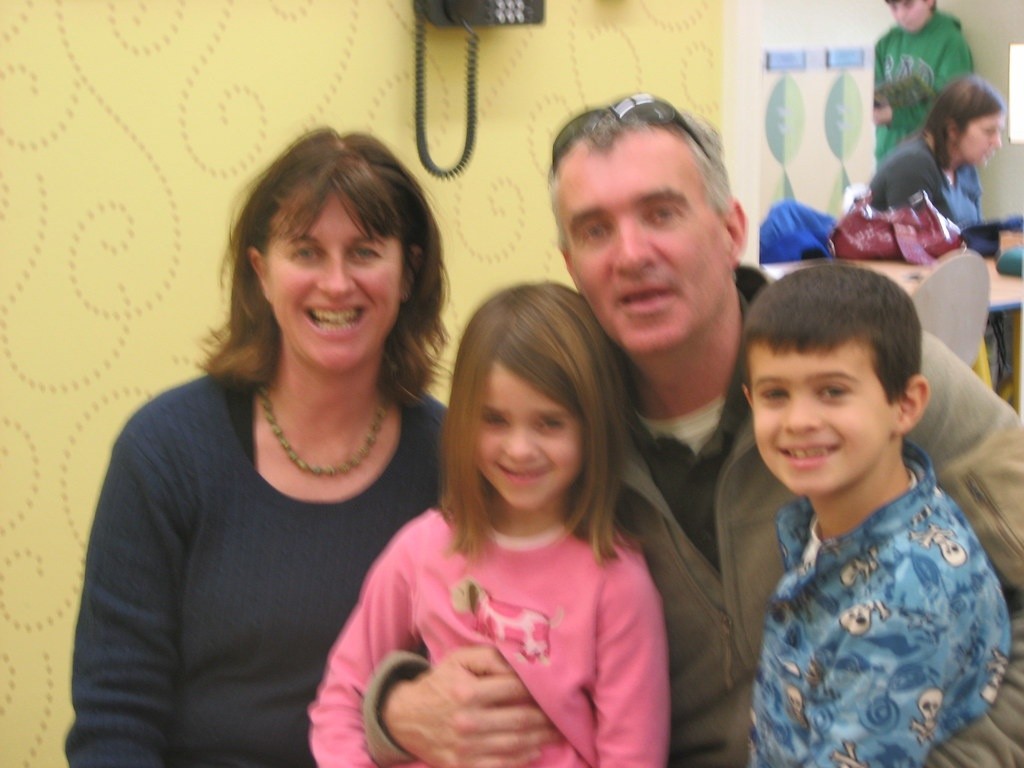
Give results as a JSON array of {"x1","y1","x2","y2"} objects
[{"x1": 827, "y1": 191, "x2": 963, "y2": 265}]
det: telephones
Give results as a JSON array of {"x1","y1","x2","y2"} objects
[{"x1": 417, "y1": 0, "x2": 544, "y2": 26}]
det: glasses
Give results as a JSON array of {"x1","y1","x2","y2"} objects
[{"x1": 552, "y1": 92, "x2": 710, "y2": 182}]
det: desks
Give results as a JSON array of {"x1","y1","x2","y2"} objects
[{"x1": 753, "y1": 228, "x2": 1024, "y2": 417}]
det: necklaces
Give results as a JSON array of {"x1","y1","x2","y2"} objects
[{"x1": 260, "y1": 385, "x2": 390, "y2": 475}]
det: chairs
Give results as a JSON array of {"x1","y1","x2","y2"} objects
[{"x1": 907, "y1": 247, "x2": 991, "y2": 369}]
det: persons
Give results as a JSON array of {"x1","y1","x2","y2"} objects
[
  {"x1": 874, "y1": 0, "x2": 974, "y2": 171},
  {"x1": 738, "y1": 266, "x2": 1011, "y2": 768},
  {"x1": 305, "y1": 280, "x2": 671, "y2": 768},
  {"x1": 64, "y1": 125, "x2": 451, "y2": 768},
  {"x1": 361, "y1": 96, "x2": 1024, "y2": 768},
  {"x1": 867, "y1": 78, "x2": 1006, "y2": 232}
]
